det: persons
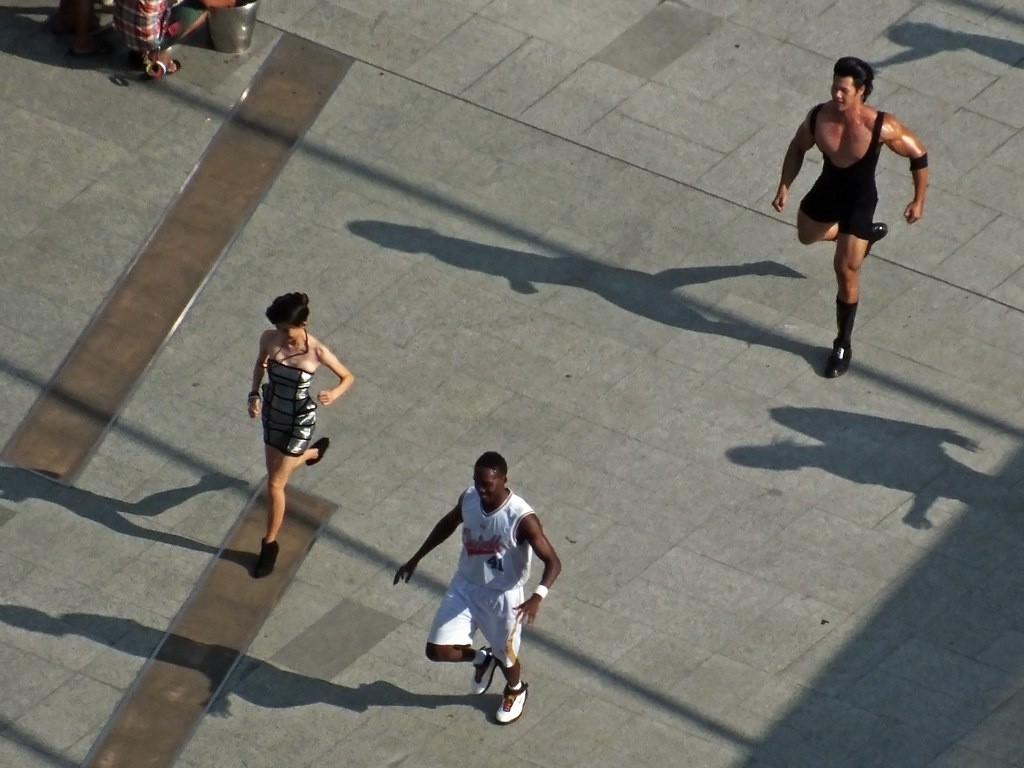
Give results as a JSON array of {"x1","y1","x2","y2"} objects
[
  {"x1": 773, "y1": 56, "x2": 929, "y2": 379},
  {"x1": 247, "y1": 291, "x2": 354, "y2": 579},
  {"x1": 54, "y1": 0, "x2": 112, "y2": 56},
  {"x1": 395, "y1": 452, "x2": 562, "y2": 725}
]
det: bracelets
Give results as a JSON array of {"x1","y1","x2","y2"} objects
[{"x1": 533, "y1": 585, "x2": 549, "y2": 599}]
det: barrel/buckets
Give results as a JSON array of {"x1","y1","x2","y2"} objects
[{"x1": 209, "y1": 1, "x2": 258, "y2": 52}]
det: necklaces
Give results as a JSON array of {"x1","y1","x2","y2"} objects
[{"x1": 260, "y1": 328, "x2": 308, "y2": 373}]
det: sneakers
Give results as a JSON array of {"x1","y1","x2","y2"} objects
[
  {"x1": 467, "y1": 647, "x2": 498, "y2": 696},
  {"x1": 824, "y1": 340, "x2": 852, "y2": 378},
  {"x1": 865, "y1": 223, "x2": 889, "y2": 256},
  {"x1": 496, "y1": 681, "x2": 529, "y2": 722},
  {"x1": 252, "y1": 538, "x2": 277, "y2": 580},
  {"x1": 308, "y1": 437, "x2": 329, "y2": 465}
]
